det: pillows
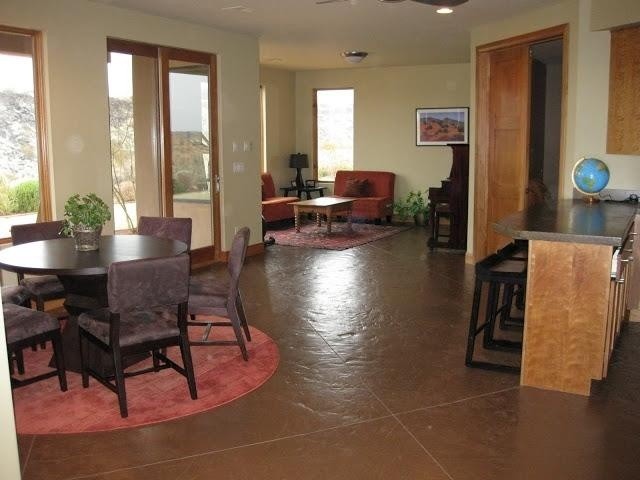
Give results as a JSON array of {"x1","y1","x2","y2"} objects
[{"x1": 340, "y1": 175, "x2": 369, "y2": 198}]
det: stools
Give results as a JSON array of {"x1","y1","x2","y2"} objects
[{"x1": 464, "y1": 240, "x2": 529, "y2": 376}]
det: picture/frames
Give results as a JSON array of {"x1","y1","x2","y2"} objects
[
  {"x1": 291, "y1": 181, "x2": 297, "y2": 187},
  {"x1": 305, "y1": 179, "x2": 315, "y2": 188},
  {"x1": 415, "y1": 106, "x2": 470, "y2": 146}
]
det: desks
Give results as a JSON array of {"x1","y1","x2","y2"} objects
[{"x1": 0, "y1": 234, "x2": 187, "y2": 352}]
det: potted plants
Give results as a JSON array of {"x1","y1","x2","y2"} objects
[
  {"x1": 58, "y1": 193, "x2": 111, "y2": 252},
  {"x1": 393, "y1": 191, "x2": 431, "y2": 226}
]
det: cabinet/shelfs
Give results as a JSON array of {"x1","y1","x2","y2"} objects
[{"x1": 520, "y1": 212, "x2": 640, "y2": 397}]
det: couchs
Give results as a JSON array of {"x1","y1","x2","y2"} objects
[
  {"x1": 261, "y1": 173, "x2": 299, "y2": 225},
  {"x1": 334, "y1": 169, "x2": 395, "y2": 225}
]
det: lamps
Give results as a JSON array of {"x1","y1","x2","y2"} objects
[
  {"x1": 290, "y1": 152, "x2": 309, "y2": 188},
  {"x1": 342, "y1": 50, "x2": 368, "y2": 66}
]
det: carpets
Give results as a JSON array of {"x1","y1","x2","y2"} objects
[
  {"x1": 12, "y1": 313, "x2": 281, "y2": 435},
  {"x1": 263, "y1": 221, "x2": 415, "y2": 251}
]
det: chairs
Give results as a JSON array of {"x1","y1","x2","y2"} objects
[
  {"x1": 78, "y1": 253, "x2": 199, "y2": 418},
  {"x1": 0, "y1": 220, "x2": 68, "y2": 397},
  {"x1": 137, "y1": 215, "x2": 197, "y2": 321},
  {"x1": 161, "y1": 226, "x2": 253, "y2": 361}
]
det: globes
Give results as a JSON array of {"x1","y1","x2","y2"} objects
[{"x1": 572, "y1": 157, "x2": 610, "y2": 203}]
